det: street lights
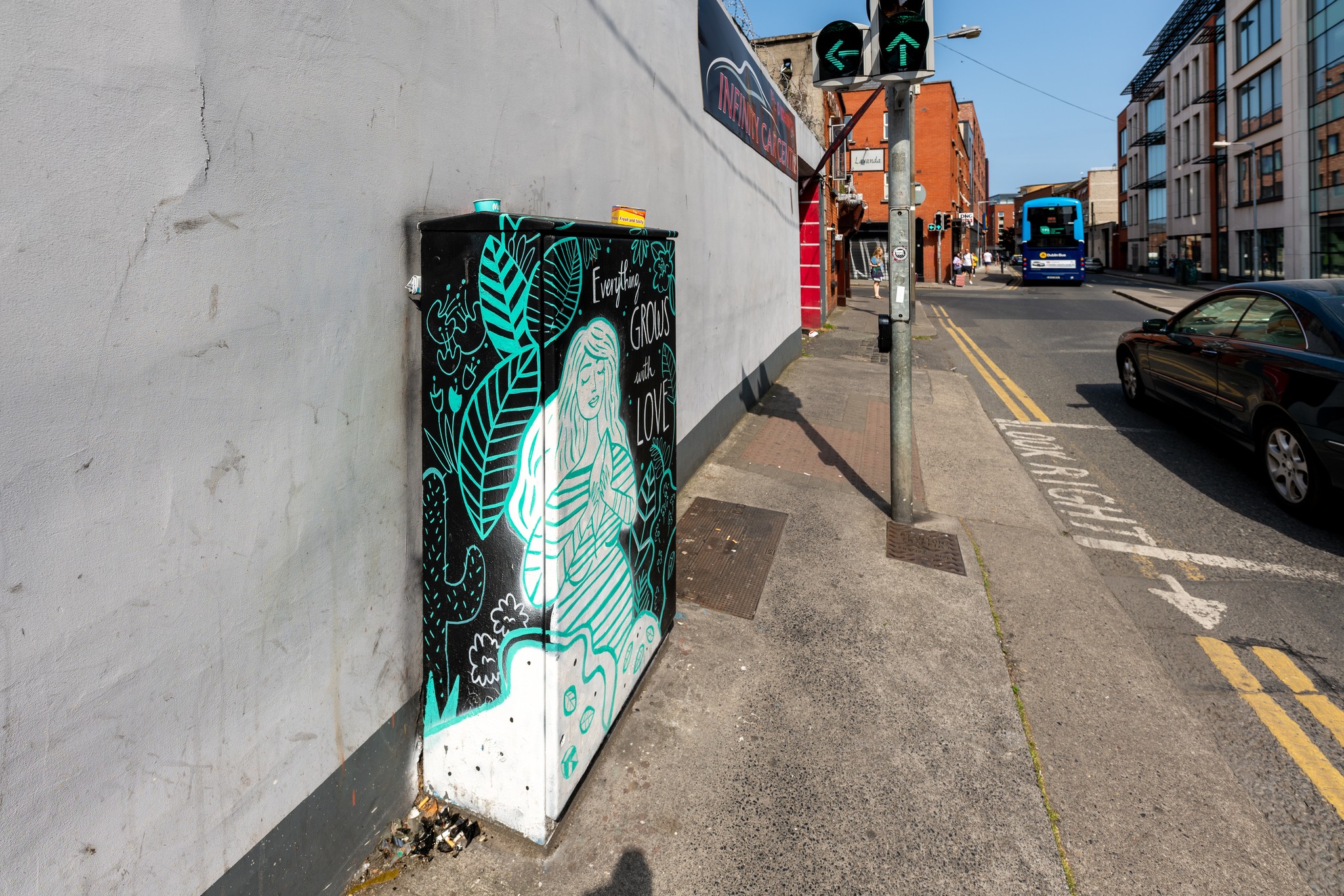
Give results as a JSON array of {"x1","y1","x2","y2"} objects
[
  {"x1": 1212, "y1": 141, "x2": 1260, "y2": 282},
  {"x1": 976, "y1": 200, "x2": 997, "y2": 269}
]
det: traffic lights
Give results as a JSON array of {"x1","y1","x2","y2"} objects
[
  {"x1": 811, "y1": 20, "x2": 872, "y2": 93},
  {"x1": 934, "y1": 213, "x2": 942, "y2": 231},
  {"x1": 869, "y1": 0, "x2": 935, "y2": 84},
  {"x1": 928, "y1": 223, "x2": 935, "y2": 231},
  {"x1": 942, "y1": 213, "x2": 951, "y2": 231}
]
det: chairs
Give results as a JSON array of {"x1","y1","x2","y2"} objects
[{"x1": 1263, "y1": 310, "x2": 1306, "y2": 348}]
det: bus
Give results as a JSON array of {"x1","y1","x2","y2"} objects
[{"x1": 1021, "y1": 197, "x2": 1086, "y2": 286}]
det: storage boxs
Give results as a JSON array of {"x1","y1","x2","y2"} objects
[{"x1": 611, "y1": 205, "x2": 646, "y2": 228}]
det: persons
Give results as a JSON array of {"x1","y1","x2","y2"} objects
[
  {"x1": 983, "y1": 249, "x2": 993, "y2": 274},
  {"x1": 980, "y1": 252, "x2": 985, "y2": 266},
  {"x1": 949, "y1": 252, "x2": 963, "y2": 285},
  {"x1": 1168, "y1": 253, "x2": 1179, "y2": 280},
  {"x1": 971, "y1": 252, "x2": 978, "y2": 277},
  {"x1": 869, "y1": 247, "x2": 885, "y2": 299},
  {"x1": 994, "y1": 252, "x2": 1000, "y2": 266},
  {"x1": 961, "y1": 248, "x2": 975, "y2": 284}
]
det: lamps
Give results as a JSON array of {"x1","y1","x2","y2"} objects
[
  {"x1": 867, "y1": 219, "x2": 872, "y2": 224},
  {"x1": 860, "y1": 200, "x2": 868, "y2": 210},
  {"x1": 865, "y1": 146, "x2": 870, "y2": 151}
]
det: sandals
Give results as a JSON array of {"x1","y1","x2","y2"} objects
[{"x1": 874, "y1": 295, "x2": 883, "y2": 299}]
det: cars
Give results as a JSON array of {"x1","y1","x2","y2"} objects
[
  {"x1": 999, "y1": 254, "x2": 1006, "y2": 262},
  {"x1": 1085, "y1": 257, "x2": 1103, "y2": 274},
  {"x1": 1115, "y1": 276, "x2": 1344, "y2": 521},
  {"x1": 1008, "y1": 254, "x2": 1022, "y2": 266}
]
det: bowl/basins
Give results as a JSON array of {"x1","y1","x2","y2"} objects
[{"x1": 473, "y1": 198, "x2": 501, "y2": 212}]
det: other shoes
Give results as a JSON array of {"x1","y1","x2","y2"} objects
[
  {"x1": 973, "y1": 274, "x2": 975, "y2": 277},
  {"x1": 969, "y1": 281, "x2": 973, "y2": 284},
  {"x1": 949, "y1": 280, "x2": 952, "y2": 285},
  {"x1": 985, "y1": 272, "x2": 990, "y2": 274}
]
